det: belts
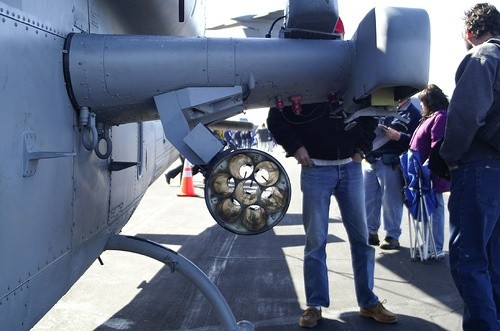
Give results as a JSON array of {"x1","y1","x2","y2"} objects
[{"x1": 375, "y1": 157, "x2": 381, "y2": 160}]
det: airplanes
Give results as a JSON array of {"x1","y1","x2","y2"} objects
[{"x1": 1, "y1": 0, "x2": 432, "y2": 331}]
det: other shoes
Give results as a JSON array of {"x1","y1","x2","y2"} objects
[
  {"x1": 368, "y1": 233, "x2": 380, "y2": 246},
  {"x1": 380, "y1": 236, "x2": 399, "y2": 248},
  {"x1": 165, "y1": 173, "x2": 170, "y2": 184},
  {"x1": 417, "y1": 244, "x2": 445, "y2": 258}
]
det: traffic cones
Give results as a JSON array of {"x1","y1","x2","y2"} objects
[{"x1": 177, "y1": 155, "x2": 202, "y2": 197}]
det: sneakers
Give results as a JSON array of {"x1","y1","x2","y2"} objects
[
  {"x1": 300, "y1": 306, "x2": 322, "y2": 327},
  {"x1": 359, "y1": 299, "x2": 398, "y2": 323}
]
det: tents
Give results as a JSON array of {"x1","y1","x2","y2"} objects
[{"x1": 399, "y1": 148, "x2": 438, "y2": 262}]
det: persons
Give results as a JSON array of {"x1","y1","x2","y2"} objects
[
  {"x1": 165, "y1": 123, "x2": 277, "y2": 184},
  {"x1": 266, "y1": 17, "x2": 397, "y2": 328},
  {"x1": 360, "y1": 84, "x2": 450, "y2": 258},
  {"x1": 428, "y1": 2, "x2": 500, "y2": 331}
]
